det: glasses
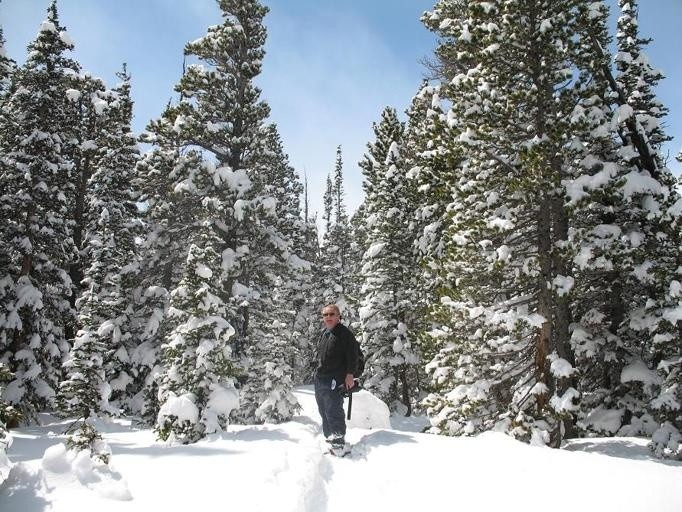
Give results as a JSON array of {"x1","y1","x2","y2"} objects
[{"x1": 322, "y1": 313, "x2": 334, "y2": 316}]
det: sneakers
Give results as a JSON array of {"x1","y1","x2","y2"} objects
[{"x1": 323, "y1": 449, "x2": 345, "y2": 459}]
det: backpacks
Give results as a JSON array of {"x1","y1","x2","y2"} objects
[{"x1": 351, "y1": 335, "x2": 364, "y2": 378}]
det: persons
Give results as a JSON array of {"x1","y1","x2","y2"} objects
[{"x1": 311, "y1": 304, "x2": 358, "y2": 458}]
